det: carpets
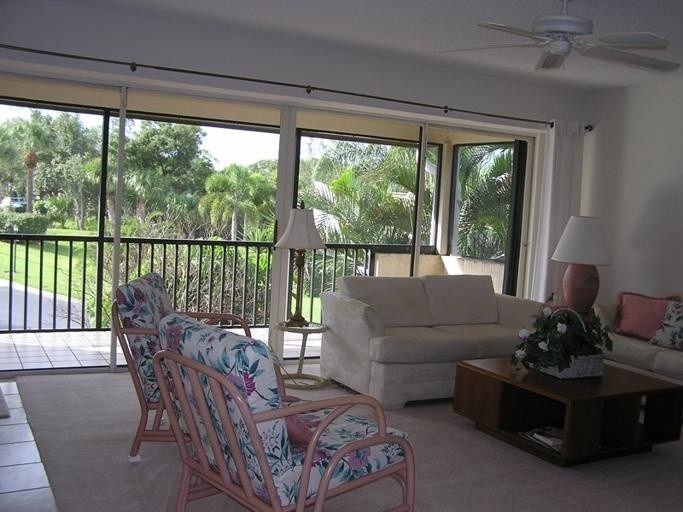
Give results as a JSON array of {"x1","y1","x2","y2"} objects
[{"x1": 9, "y1": 364, "x2": 683, "y2": 511}]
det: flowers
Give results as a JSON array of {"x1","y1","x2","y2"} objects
[{"x1": 511, "y1": 302, "x2": 614, "y2": 368}]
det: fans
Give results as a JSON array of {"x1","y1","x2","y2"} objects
[{"x1": 433, "y1": 1, "x2": 668, "y2": 72}]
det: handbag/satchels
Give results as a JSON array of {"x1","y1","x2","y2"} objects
[{"x1": 528, "y1": 307, "x2": 604, "y2": 379}]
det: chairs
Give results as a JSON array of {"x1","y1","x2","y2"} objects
[
  {"x1": 151, "y1": 308, "x2": 419, "y2": 510},
  {"x1": 109, "y1": 269, "x2": 290, "y2": 464}
]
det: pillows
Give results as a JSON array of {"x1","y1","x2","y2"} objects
[
  {"x1": 280, "y1": 402, "x2": 322, "y2": 447},
  {"x1": 610, "y1": 292, "x2": 679, "y2": 341},
  {"x1": 644, "y1": 299, "x2": 681, "y2": 352}
]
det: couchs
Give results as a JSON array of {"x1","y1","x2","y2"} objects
[
  {"x1": 594, "y1": 289, "x2": 681, "y2": 396},
  {"x1": 316, "y1": 270, "x2": 551, "y2": 416}
]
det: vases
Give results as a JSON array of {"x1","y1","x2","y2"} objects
[{"x1": 529, "y1": 348, "x2": 608, "y2": 382}]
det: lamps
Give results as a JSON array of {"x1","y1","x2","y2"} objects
[
  {"x1": 268, "y1": 206, "x2": 326, "y2": 326},
  {"x1": 551, "y1": 213, "x2": 617, "y2": 312},
  {"x1": 548, "y1": 40, "x2": 574, "y2": 58}
]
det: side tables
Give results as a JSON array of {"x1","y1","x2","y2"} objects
[{"x1": 275, "y1": 315, "x2": 332, "y2": 393}]
